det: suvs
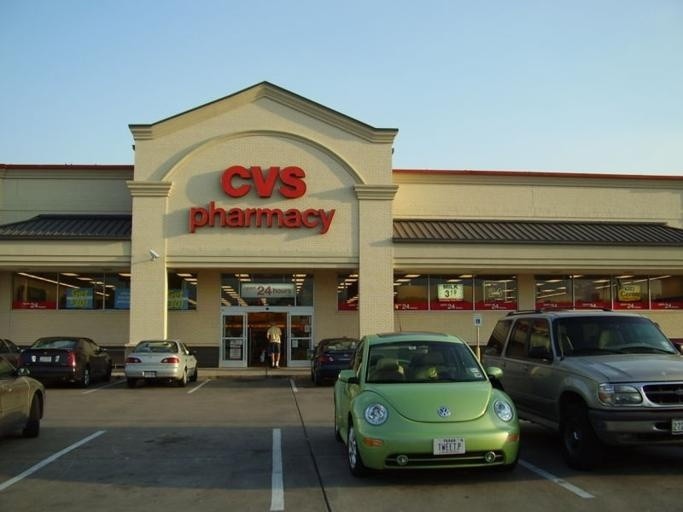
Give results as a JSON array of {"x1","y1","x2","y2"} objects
[{"x1": 481, "y1": 306, "x2": 682, "y2": 471}]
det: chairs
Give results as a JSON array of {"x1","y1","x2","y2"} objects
[
  {"x1": 596, "y1": 320, "x2": 629, "y2": 351},
  {"x1": 421, "y1": 350, "x2": 448, "y2": 378}
]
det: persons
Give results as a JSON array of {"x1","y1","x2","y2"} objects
[{"x1": 266, "y1": 319, "x2": 283, "y2": 368}]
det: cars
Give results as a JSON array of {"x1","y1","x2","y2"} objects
[
  {"x1": 1, "y1": 338, "x2": 46, "y2": 437},
  {"x1": 124, "y1": 339, "x2": 198, "y2": 386},
  {"x1": 309, "y1": 338, "x2": 361, "y2": 385},
  {"x1": 332, "y1": 330, "x2": 520, "y2": 475},
  {"x1": 21, "y1": 335, "x2": 112, "y2": 388}
]
